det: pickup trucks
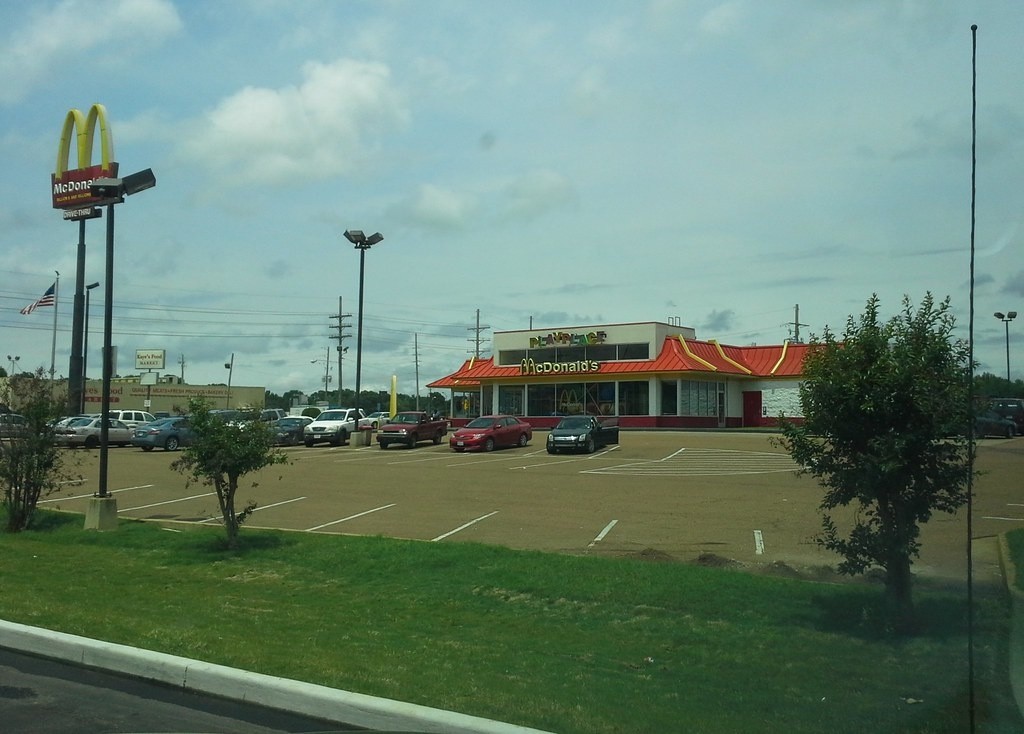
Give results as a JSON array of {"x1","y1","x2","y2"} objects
[{"x1": 376, "y1": 410, "x2": 448, "y2": 449}]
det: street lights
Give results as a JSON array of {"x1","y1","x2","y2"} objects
[
  {"x1": 81, "y1": 282, "x2": 100, "y2": 414},
  {"x1": 343, "y1": 229, "x2": 384, "y2": 431},
  {"x1": 224, "y1": 353, "x2": 234, "y2": 410},
  {"x1": 994, "y1": 311, "x2": 1018, "y2": 382},
  {"x1": 310, "y1": 346, "x2": 330, "y2": 400}
]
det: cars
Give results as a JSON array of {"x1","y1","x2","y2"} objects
[
  {"x1": 449, "y1": 414, "x2": 533, "y2": 452},
  {"x1": 971, "y1": 407, "x2": 1019, "y2": 440},
  {"x1": 0, "y1": 414, "x2": 37, "y2": 439},
  {"x1": 546, "y1": 414, "x2": 620, "y2": 454},
  {"x1": 128, "y1": 416, "x2": 197, "y2": 452},
  {"x1": 52, "y1": 417, "x2": 135, "y2": 449},
  {"x1": 267, "y1": 416, "x2": 314, "y2": 446},
  {"x1": 365, "y1": 411, "x2": 400, "y2": 430}
]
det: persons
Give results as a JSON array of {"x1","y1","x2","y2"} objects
[
  {"x1": 431, "y1": 409, "x2": 440, "y2": 420},
  {"x1": 464, "y1": 398, "x2": 469, "y2": 411}
]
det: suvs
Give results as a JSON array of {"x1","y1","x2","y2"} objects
[
  {"x1": 987, "y1": 398, "x2": 1024, "y2": 435},
  {"x1": 303, "y1": 407, "x2": 370, "y2": 447},
  {"x1": 96, "y1": 409, "x2": 158, "y2": 430},
  {"x1": 204, "y1": 407, "x2": 288, "y2": 442}
]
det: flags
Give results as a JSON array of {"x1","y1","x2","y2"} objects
[{"x1": 20, "y1": 282, "x2": 54, "y2": 315}]
